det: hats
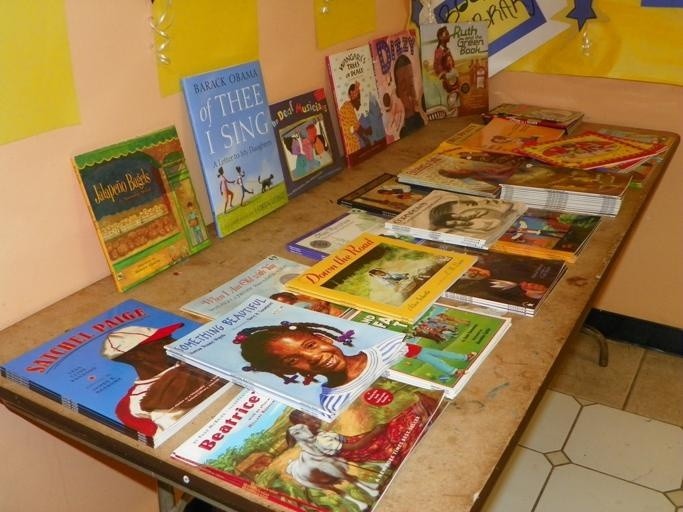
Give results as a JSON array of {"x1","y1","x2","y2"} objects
[{"x1": 103, "y1": 323, "x2": 185, "y2": 359}]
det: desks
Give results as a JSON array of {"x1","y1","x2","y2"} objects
[{"x1": 0, "y1": 106, "x2": 681, "y2": 511}]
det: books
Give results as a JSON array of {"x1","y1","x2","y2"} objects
[
  {"x1": 1, "y1": 255, "x2": 449, "y2": 512},
  {"x1": 270, "y1": 88, "x2": 347, "y2": 199},
  {"x1": 384, "y1": 100, "x2": 670, "y2": 318},
  {"x1": 70, "y1": 125, "x2": 211, "y2": 294},
  {"x1": 285, "y1": 171, "x2": 513, "y2": 403},
  {"x1": 419, "y1": 21, "x2": 490, "y2": 120},
  {"x1": 181, "y1": 60, "x2": 289, "y2": 239},
  {"x1": 325, "y1": 28, "x2": 430, "y2": 168}
]
